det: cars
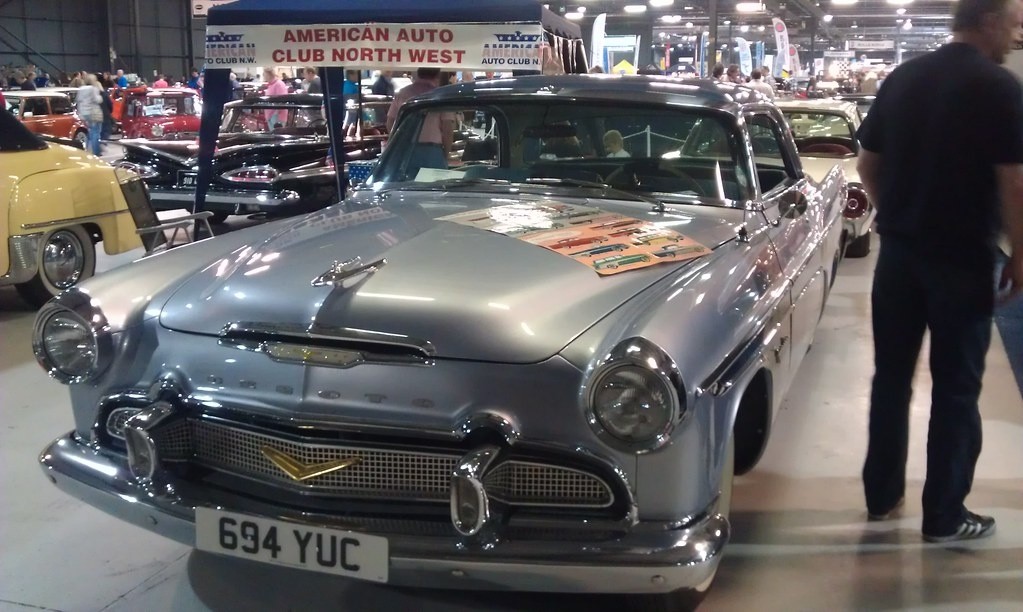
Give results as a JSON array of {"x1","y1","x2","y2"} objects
[
  {"x1": 114, "y1": 91, "x2": 483, "y2": 226},
  {"x1": 119, "y1": 87, "x2": 202, "y2": 146},
  {"x1": 35, "y1": 86, "x2": 152, "y2": 135},
  {"x1": 31, "y1": 76, "x2": 851, "y2": 607},
  {"x1": 0, "y1": 100, "x2": 153, "y2": 308},
  {"x1": 2, "y1": 91, "x2": 89, "y2": 150},
  {"x1": 659, "y1": 98, "x2": 878, "y2": 259}
]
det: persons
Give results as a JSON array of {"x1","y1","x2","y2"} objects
[
  {"x1": 0, "y1": 64, "x2": 204, "y2": 157},
  {"x1": 524, "y1": 119, "x2": 632, "y2": 159},
  {"x1": 226, "y1": 66, "x2": 494, "y2": 182},
  {"x1": 855, "y1": 0, "x2": 1023, "y2": 544},
  {"x1": 712, "y1": 63, "x2": 855, "y2": 100}
]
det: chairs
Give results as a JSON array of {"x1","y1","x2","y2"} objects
[
  {"x1": 114, "y1": 161, "x2": 214, "y2": 258},
  {"x1": 799, "y1": 136, "x2": 856, "y2": 154},
  {"x1": 751, "y1": 136, "x2": 778, "y2": 152}
]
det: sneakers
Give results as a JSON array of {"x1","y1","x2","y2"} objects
[
  {"x1": 865, "y1": 488, "x2": 903, "y2": 519},
  {"x1": 922, "y1": 508, "x2": 995, "y2": 541}
]
